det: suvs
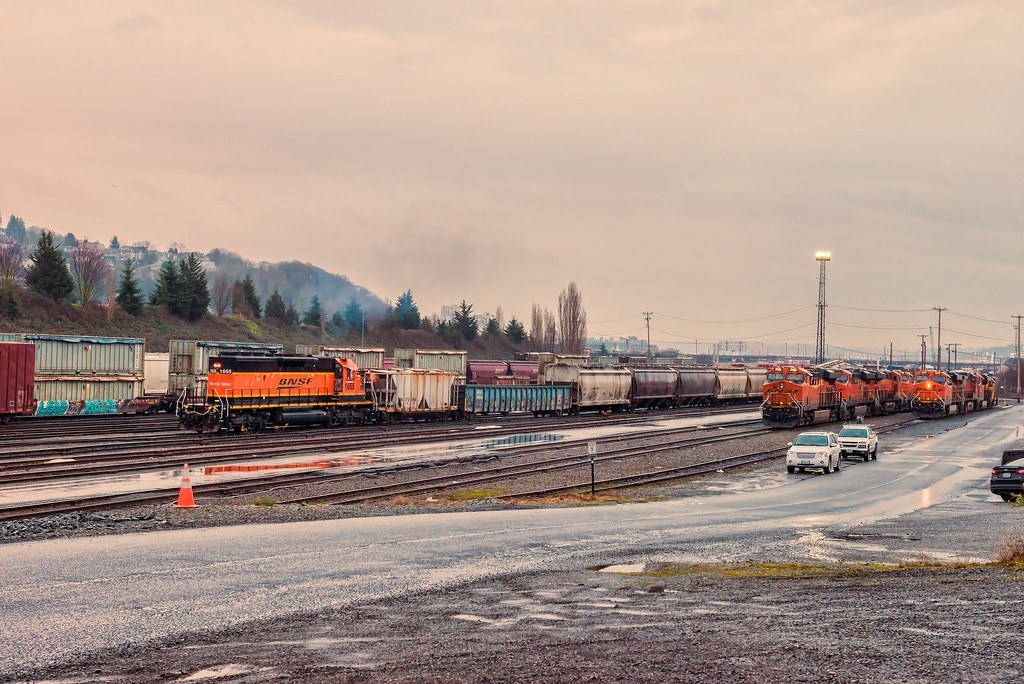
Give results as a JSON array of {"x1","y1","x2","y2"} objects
[{"x1": 785, "y1": 431, "x2": 841, "y2": 475}]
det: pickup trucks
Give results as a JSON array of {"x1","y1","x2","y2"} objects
[{"x1": 836, "y1": 423, "x2": 878, "y2": 463}]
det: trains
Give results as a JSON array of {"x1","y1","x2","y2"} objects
[
  {"x1": 0, "y1": 332, "x2": 792, "y2": 437},
  {"x1": 758, "y1": 362, "x2": 936, "y2": 429},
  {"x1": 909, "y1": 367, "x2": 1000, "y2": 420}
]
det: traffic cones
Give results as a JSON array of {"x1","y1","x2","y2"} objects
[{"x1": 172, "y1": 463, "x2": 201, "y2": 508}]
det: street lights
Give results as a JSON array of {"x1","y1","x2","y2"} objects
[{"x1": 814, "y1": 250, "x2": 830, "y2": 364}]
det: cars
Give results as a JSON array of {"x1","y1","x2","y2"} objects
[{"x1": 990, "y1": 449, "x2": 1024, "y2": 503}]
